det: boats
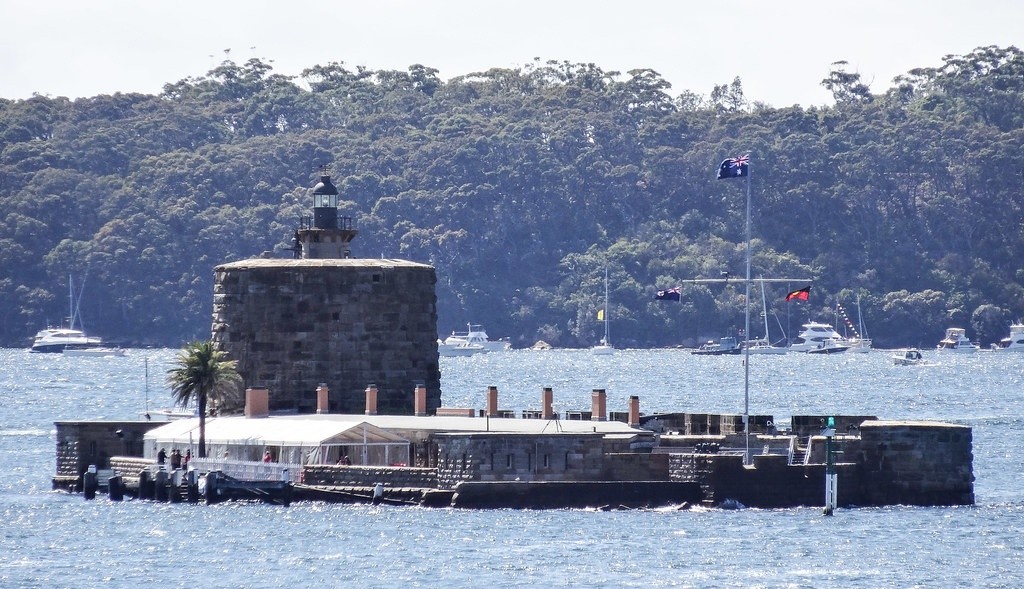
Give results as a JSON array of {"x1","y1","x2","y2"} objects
[
  {"x1": 892, "y1": 349, "x2": 931, "y2": 365},
  {"x1": 438, "y1": 321, "x2": 513, "y2": 358},
  {"x1": 808, "y1": 338, "x2": 851, "y2": 355},
  {"x1": 450, "y1": 341, "x2": 491, "y2": 357},
  {"x1": 934, "y1": 328, "x2": 980, "y2": 354},
  {"x1": 529, "y1": 340, "x2": 553, "y2": 351},
  {"x1": 62, "y1": 344, "x2": 125, "y2": 357},
  {"x1": 690, "y1": 337, "x2": 740, "y2": 355},
  {"x1": 743, "y1": 344, "x2": 790, "y2": 355},
  {"x1": 995, "y1": 324, "x2": 1023, "y2": 352}
]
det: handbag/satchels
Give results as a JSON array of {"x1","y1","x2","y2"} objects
[{"x1": 182, "y1": 463, "x2": 187, "y2": 470}]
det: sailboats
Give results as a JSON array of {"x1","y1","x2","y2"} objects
[
  {"x1": 591, "y1": 267, "x2": 616, "y2": 356},
  {"x1": 30, "y1": 274, "x2": 104, "y2": 353},
  {"x1": 784, "y1": 294, "x2": 872, "y2": 353},
  {"x1": 740, "y1": 274, "x2": 797, "y2": 348}
]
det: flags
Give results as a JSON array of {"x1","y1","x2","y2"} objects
[
  {"x1": 785, "y1": 285, "x2": 811, "y2": 303},
  {"x1": 654, "y1": 286, "x2": 682, "y2": 303},
  {"x1": 716, "y1": 154, "x2": 749, "y2": 180}
]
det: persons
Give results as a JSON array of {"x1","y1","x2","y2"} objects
[
  {"x1": 264, "y1": 451, "x2": 271, "y2": 462},
  {"x1": 337, "y1": 455, "x2": 351, "y2": 465},
  {"x1": 158, "y1": 448, "x2": 190, "y2": 471}
]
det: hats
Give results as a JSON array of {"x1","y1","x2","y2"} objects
[
  {"x1": 161, "y1": 447, "x2": 165, "y2": 451},
  {"x1": 266, "y1": 451, "x2": 270, "y2": 453},
  {"x1": 345, "y1": 456, "x2": 349, "y2": 459}
]
